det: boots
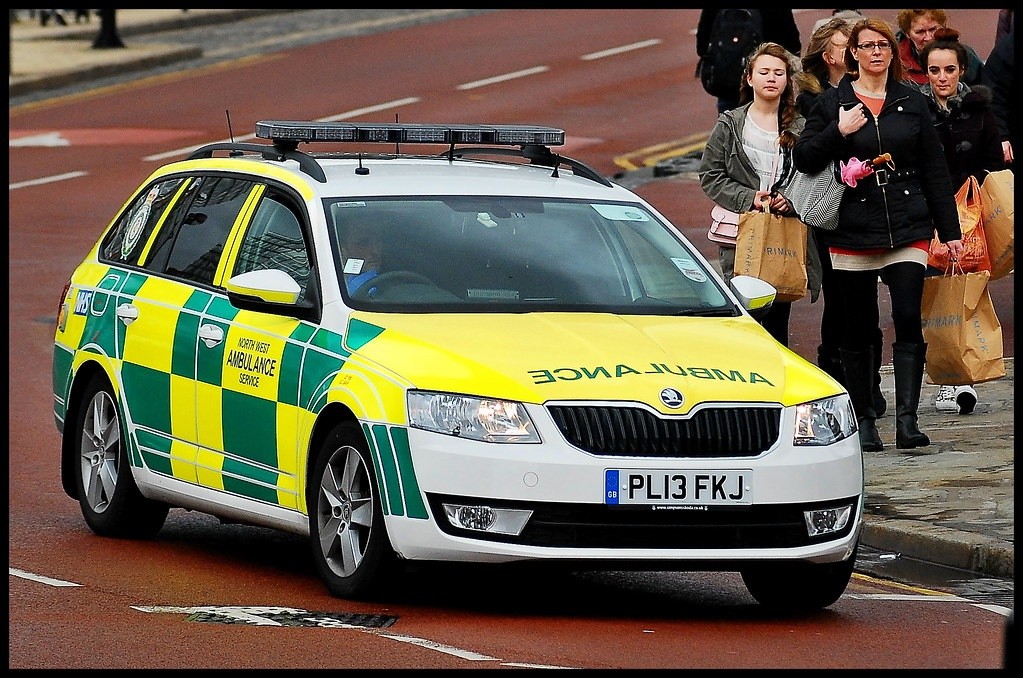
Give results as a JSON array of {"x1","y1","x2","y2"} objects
[
  {"x1": 839, "y1": 341, "x2": 883, "y2": 452},
  {"x1": 893, "y1": 342, "x2": 930, "y2": 449}
]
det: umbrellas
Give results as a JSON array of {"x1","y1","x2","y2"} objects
[{"x1": 836, "y1": 154, "x2": 892, "y2": 189}]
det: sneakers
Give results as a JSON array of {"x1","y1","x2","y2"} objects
[
  {"x1": 955, "y1": 385, "x2": 978, "y2": 415},
  {"x1": 936, "y1": 386, "x2": 958, "y2": 413}
]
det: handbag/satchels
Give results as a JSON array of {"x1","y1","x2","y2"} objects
[
  {"x1": 733, "y1": 199, "x2": 808, "y2": 302},
  {"x1": 783, "y1": 87, "x2": 846, "y2": 230},
  {"x1": 981, "y1": 168, "x2": 1014, "y2": 281},
  {"x1": 929, "y1": 176, "x2": 992, "y2": 273},
  {"x1": 706, "y1": 204, "x2": 741, "y2": 248},
  {"x1": 923, "y1": 254, "x2": 1005, "y2": 384}
]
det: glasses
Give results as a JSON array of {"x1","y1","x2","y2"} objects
[{"x1": 857, "y1": 41, "x2": 891, "y2": 49}]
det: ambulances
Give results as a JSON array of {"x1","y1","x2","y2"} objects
[{"x1": 50, "y1": 119, "x2": 866, "y2": 614}]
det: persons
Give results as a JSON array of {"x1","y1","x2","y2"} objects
[
  {"x1": 693, "y1": 6, "x2": 1014, "y2": 451},
  {"x1": 336, "y1": 213, "x2": 384, "y2": 274}
]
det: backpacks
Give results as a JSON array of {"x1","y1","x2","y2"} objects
[{"x1": 700, "y1": 10, "x2": 765, "y2": 103}]
